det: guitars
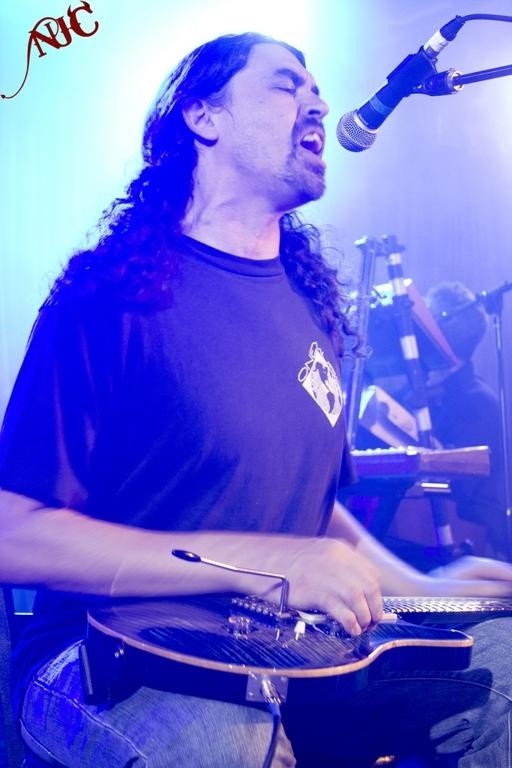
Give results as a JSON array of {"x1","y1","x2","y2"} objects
[{"x1": 82, "y1": 592, "x2": 511, "y2": 709}]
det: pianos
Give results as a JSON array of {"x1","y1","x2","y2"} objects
[
  {"x1": 347, "y1": 444, "x2": 490, "y2": 476},
  {"x1": 341, "y1": 279, "x2": 455, "y2": 377}
]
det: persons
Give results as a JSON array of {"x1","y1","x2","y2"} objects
[
  {"x1": 2, "y1": 28, "x2": 511, "y2": 766},
  {"x1": 419, "y1": 278, "x2": 511, "y2": 565}
]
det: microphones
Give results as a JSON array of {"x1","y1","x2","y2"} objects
[{"x1": 336, "y1": 15, "x2": 464, "y2": 152}]
